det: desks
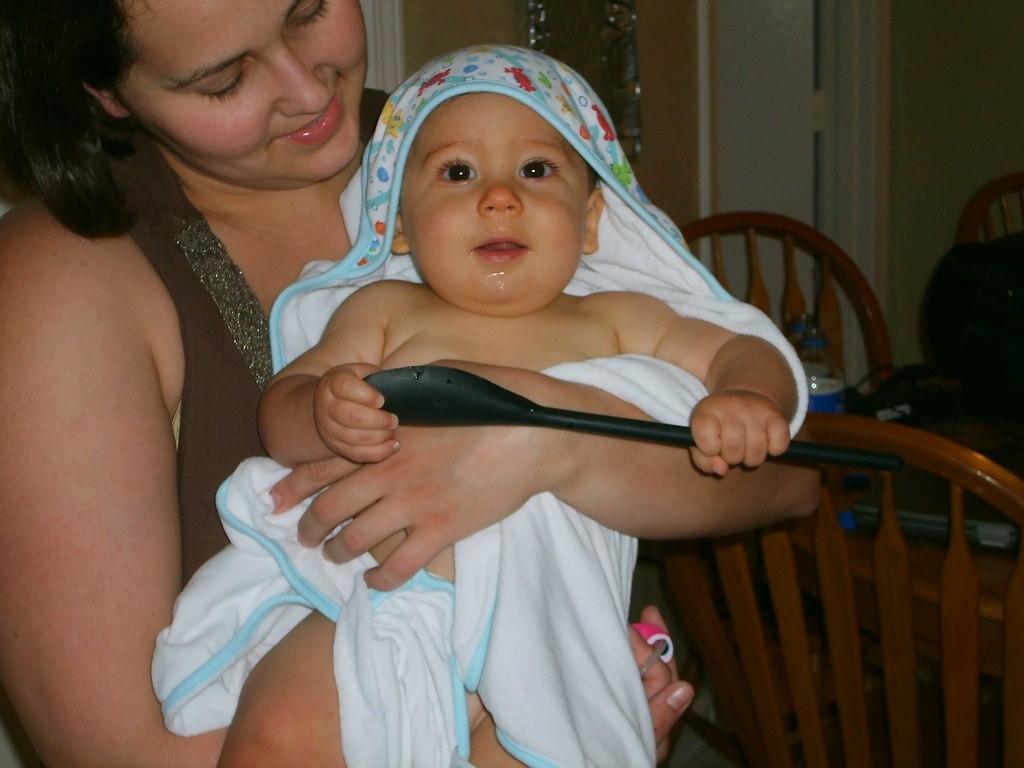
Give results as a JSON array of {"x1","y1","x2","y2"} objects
[{"x1": 775, "y1": 414, "x2": 1024, "y2": 682}]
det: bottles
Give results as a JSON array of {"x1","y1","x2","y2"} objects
[{"x1": 783, "y1": 314, "x2": 860, "y2": 528}]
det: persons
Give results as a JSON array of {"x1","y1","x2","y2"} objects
[
  {"x1": 150, "y1": 42, "x2": 808, "y2": 768},
  {"x1": 0, "y1": 0, "x2": 822, "y2": 768}
]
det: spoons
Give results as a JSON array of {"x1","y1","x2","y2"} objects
[{"x1": 359, "y1": 365, "x2": 904, "y2": 474}]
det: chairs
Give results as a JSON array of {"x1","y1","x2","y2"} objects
[
  {"x1": 680, "y1": 211, "x2": 893, "y2": 401},
  {"x1": 955, "y1": 173, "x2": 1024, "y2": 246},
  {"x1": 628, "y1": 413, "x2": 1024, "y2": 768}
]
